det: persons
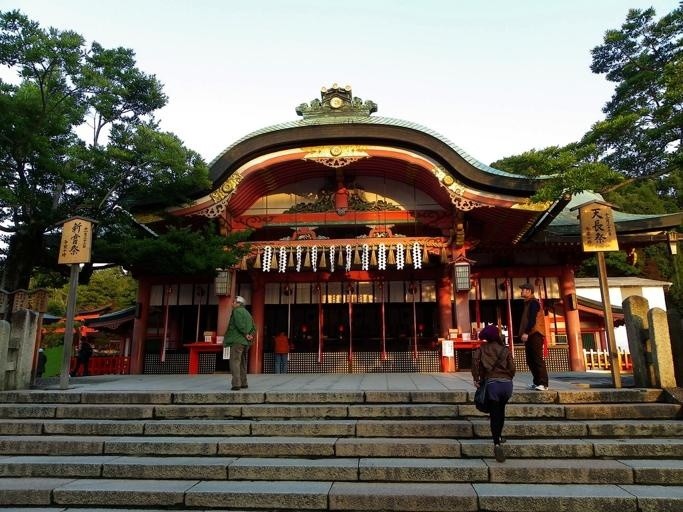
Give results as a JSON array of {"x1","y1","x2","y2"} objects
[
  {"x1": 273, "y1": 330, "x2": 290, "y2": 373},
  {"x1": 222, "y1": 295, "x2": 256, "y2": 391},
  {"x1": 470, "y1": 325, "x2": 517, "y2": 464},
  {"x1": 71, "y1": 335, "x2": 94, "y2": 377},
  {"x1": 36, "y1": 345, "x2": 48, "y2": 377},
  {"x1": 518, "y1": 283, "x2": 549, "y2": 391}
]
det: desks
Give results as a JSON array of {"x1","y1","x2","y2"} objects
[
  {"x1": 451, "y1": 336, "x2": 489, "y2": 372},
  {"x1": 185, "y1": 343, "x2": 226, "y2": 375}
]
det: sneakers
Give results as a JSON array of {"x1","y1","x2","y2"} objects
[
  {"x1": 528, "y1": 384, "x2": 549, "y2": 391},
  {"x1": 493, "y1": 436, "x2": 506, "y2": 462},
  {"x1": 231, "y1": 385, "x2": 248, "y2": 391}
]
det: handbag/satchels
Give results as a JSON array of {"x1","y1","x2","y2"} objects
[{"x1": 474, "y1": 377, "x2": 490, "y2": 413}]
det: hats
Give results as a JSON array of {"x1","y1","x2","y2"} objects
[
  {"x1": 519, "y1": 283, "x2": 534, "y2": 289},
  {"x1": 478, "y1": 325, "x2": 500, "y2": 343}
]
